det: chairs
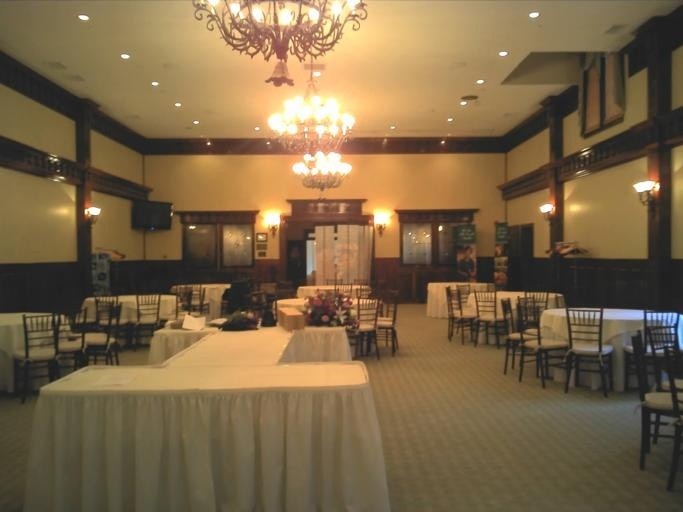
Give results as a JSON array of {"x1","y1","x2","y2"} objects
[
  {"x1": 633, "y1": 330, "x2": 680, "y2": 491},
  {"x1": 445, "y1": 282, "x2": 569, "y2": 348},
  {"x1": 501, "y1": 296, "x2": 682, "y2": 398},
  {"x1": 0, "y1": 258, "x2": 398, "y2": 402}
]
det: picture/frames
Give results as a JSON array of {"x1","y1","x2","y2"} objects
[{"x1": 255, "y1": 232, "x2": 268, "y2": 243}]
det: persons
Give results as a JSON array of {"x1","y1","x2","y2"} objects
[
  {"x1": 456, "y1": 245, "x2": 475, "y2": 282},
  {"x1": 495, "y1": 243, "x2": 503, "y2": 258}
]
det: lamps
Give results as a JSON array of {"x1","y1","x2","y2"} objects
[
  {"x1": 184, "y1": 1, "x2": 369, "y2": 86},
  {"x1": 632, "y1": 179, "x2": 661, "y2": 208},
  {"x1": 289, "y1": 151, "x2": 354, "y2": 190},
  {"x1": 538, "y1": 202, "x2": 556, "y2": 221},
  {"x1": 265, "y1": 212, "x2": 280, "y2": 237},
  {"x1": 87, "y1": 206, "x2": 102, "y2": 223},
  {"x1": 264, "y1": 49, "x2": 359, "y2": 159},
  {"x1": 372, "y1": 211, "x2": 389, "y2": 236}
]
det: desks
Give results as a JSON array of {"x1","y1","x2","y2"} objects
[
  {"x1": 39, "y1": 361, "x2": 371, "y2": 511},
  {"x1": 426, "y1": 282, "x2": 496, "y2": 319}
]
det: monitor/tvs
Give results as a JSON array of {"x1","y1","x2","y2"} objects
[{"x1": 130, "y1": 199, "x2": 172, "y2": 231}]
